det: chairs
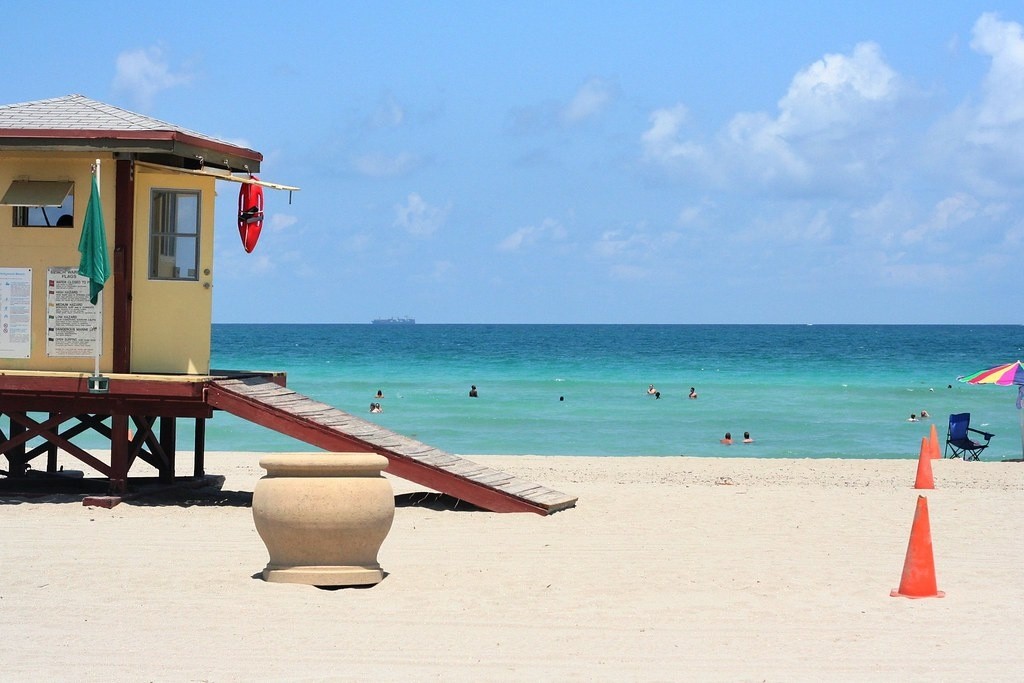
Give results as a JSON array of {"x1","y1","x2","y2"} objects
[{"x1": 944, "y1": 412, "x2": 995, "y2": 461}]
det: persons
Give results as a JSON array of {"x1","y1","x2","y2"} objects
[
  {"x1": 647, "y1": 384, "x2": 661, "y2": 400},
  {"x1": 374, "y1": 390, "x2": 384, "y2": 398},
  {"x1": 919, "y1": 409, "x2": 930, "y2": 417},
  {"x1": 560, "y1": 396, "x2": 564, "y2": 401},
  {"x1": 369, "y1": 402, "x2": 383, "y2": 413},
  {"x1": 689, "y1": 387, "x2": 697, "y2": 400},
  {"x1": 720, "y1": 432, "x2": 734, "y2": 444},
  {"x1": 742, "y1": 432, "x2": 754, "y2": 443},
  {"x1": 906, "y1": 413, "x2": 919, "y2": 422},
  {"x1": 469, "y1": 385, "x2": 479, "y2": 397}
]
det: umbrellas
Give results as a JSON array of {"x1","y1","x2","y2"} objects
[{"x1": 955, "y1": 359, "x2": 1024, "y2": 409}]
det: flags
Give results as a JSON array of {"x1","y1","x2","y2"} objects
[{"x1": 78, "y1": 172, "x2": 111, "y2": 306}]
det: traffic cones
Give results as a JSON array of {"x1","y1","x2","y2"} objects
[
  {"x1": 889, "y1": 495, "x2": 946, "y2": 599},
  {"x1": 912, "y1": 436, "x2": 937, "y2": 490},
  {"x1": 927, "y1": 423, "x2": 942, "y2": 459}
]
